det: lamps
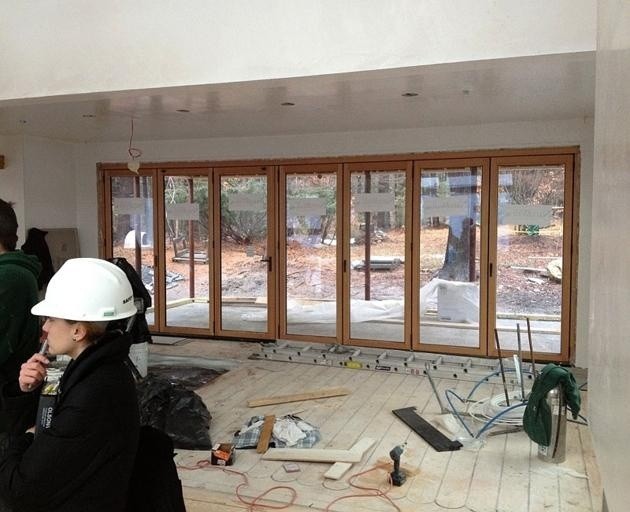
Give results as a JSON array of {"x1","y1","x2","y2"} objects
[{"x1": 127, "y1": 115, "x2": 143, "y2": 176}]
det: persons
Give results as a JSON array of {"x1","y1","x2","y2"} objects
[
  {"x1": 0, "y1": 257, "x2": 138, "y2": 511},
  {"x1": 0, "y1": 198, "x2": 43, "y2": 390}
]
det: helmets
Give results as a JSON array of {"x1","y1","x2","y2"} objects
[{"x1": 30, "y1": 257, "x2": 137, "y2": 323}]
